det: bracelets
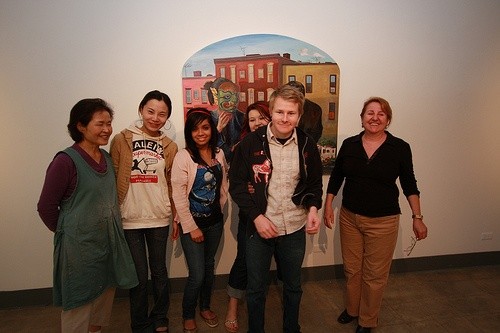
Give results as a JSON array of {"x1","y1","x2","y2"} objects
[{"x1": 412, "y1": 214, "x2": 423, "y2": 220}]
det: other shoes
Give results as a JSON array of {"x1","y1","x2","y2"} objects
[
  {"x1": 199, "y1": 309, "x2": 220, "y2": 328},
  {"x1": 337, "y1": 308, "x2": 358, "y2": 324},
  {"x1": 183, "y1": 325, "x2": 198, "y2": 333},
  {"x1": 355, "y1": 324, "x2": 372, "y2": 333}
]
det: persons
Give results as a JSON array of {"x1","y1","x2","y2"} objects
[
  {"x1": 37, "y1": 99, "x2": 138, "y2": 333},
  {"x1": 109, "y1": 90, "x2": 180, "y2": 333},
  {"x1": 224, "y1": 101, "x2": 271, "y2": 333},
  {"x1": 227, "y1": 88, "x2": 324, "y2": 333},
  {"x1": 170, "y1": 108, "x2": 230, "y2": 333},
  {"x1": 324, "y1": 97, "x2": 427, "y2": 333}
]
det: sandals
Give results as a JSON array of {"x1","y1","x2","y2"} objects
[{"x1": 225, "y1": 319, "x2": 240, "y2": 333}]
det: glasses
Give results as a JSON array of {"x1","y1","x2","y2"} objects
[{"x1": 402, "y1": 236, "x2": 417, "y2": 257}]
